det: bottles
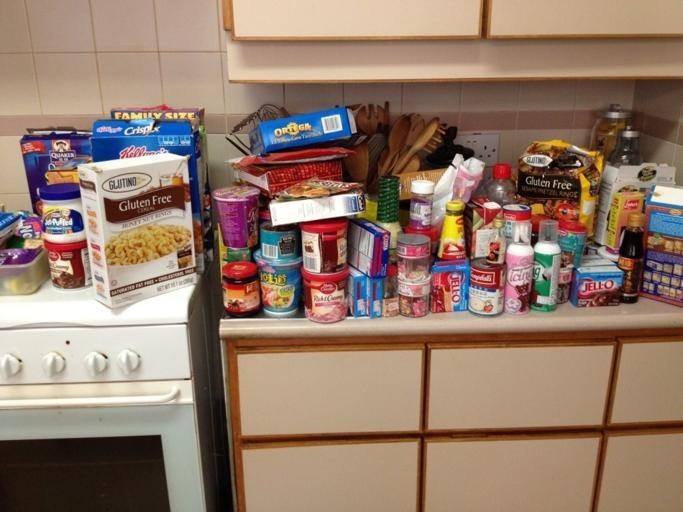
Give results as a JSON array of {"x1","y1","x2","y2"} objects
[
  {"x1": 589, "y1": 103, "x2": 641, "y2": 247},
  {"x1": 615, "y1": 212, "x2": 645, "y2": 305},
  {"x1": 533, "y1": 218, "x2": 562, "y2": 312},
  {"x1": 436, "y1": 199, "x2": 467, "y2": 260},
  {"x1": 504, "y1": 221, "x2": 534, "y2": 313},
  {"x1": 376, "y1": 174, "x2": 403, "y2": 263},
  {"x1": 408, "y1": 180, "x2": 435, "y2": 231},
  {"x1": 485, "y1": 161, "x2": 517, "y2": 205}
]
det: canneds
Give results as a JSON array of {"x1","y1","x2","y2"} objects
[
  {"x1": 209, "y1": 183, "x2": 350, "y2": 324},
  {"x1": 469, "y1": 259, "x2": 505, "y2": 315}
]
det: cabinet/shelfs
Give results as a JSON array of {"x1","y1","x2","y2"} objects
[
  {"x1": 427, "y1": 338, "x2": 599, "y2": 512},
  {"x1": 222, "y1": 0, "x2": 683, "y2": 83},
  {"x1": 601, "y1": 338, "x2": 682, "y2": 510},
  {"x1": 224, "y1": 338, "x2": 425, "y2": 512}
]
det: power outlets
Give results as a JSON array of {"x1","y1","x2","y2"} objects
[{"x1": 454, "y1": 133, "x2": 499, "y2": 165}]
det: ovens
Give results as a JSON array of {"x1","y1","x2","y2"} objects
[{"x1": 1, "y1": 322, "x2": 209, "y2": 512}]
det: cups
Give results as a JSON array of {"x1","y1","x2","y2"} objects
[{"x1": 159, "y1": 173, "x2": 183, "y2": 189}]
